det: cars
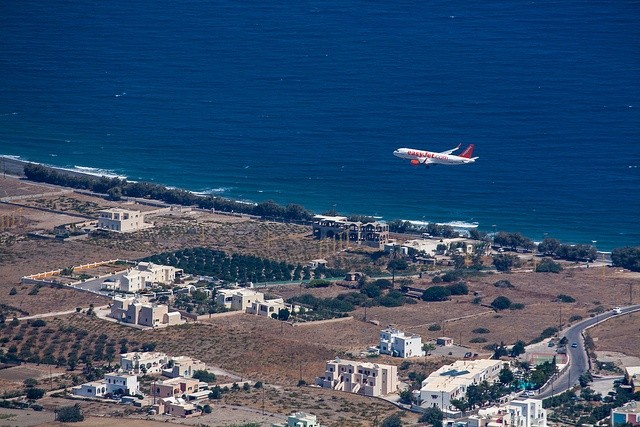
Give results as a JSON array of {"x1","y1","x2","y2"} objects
[{"x1": 571, "y1": 342, "x2": 577, "y2": 347}]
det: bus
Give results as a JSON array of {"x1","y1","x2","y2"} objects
[{"x1": 612, "y1": 306, "x2": 621, "y2": 314}]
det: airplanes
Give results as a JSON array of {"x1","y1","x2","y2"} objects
[{"x1": 393, "y1": 142, "x2": 479, "y2": 168}]
